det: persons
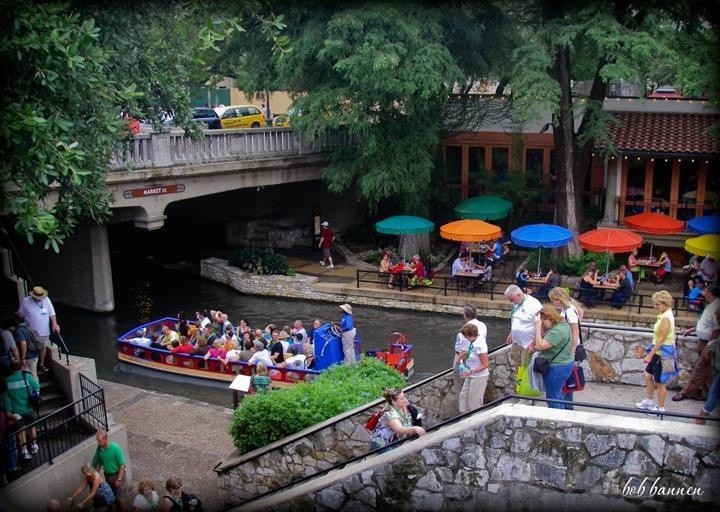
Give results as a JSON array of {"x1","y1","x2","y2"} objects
[
  {"x1": 629, "y1": 250, "x2": 671, "y2": 283},
  {"x1": 459, "y1": 324, "x2": 489, "y2": 412},
  {"x1": 507, "y1": 284, "x2": 542, "y2": 370},
  {"x1": 673, "y1": 283, "x2": 720, "y2": 401},
  {"x1": 518, "y1": 268, "x2": 560, "y2": 295},
  {"x1": 635, "y1": 291, "x2": 676, "y2": 417},
  {"x1": 2, "y1": 287, "x2": 61, "y2": 475},
  {"x1": 380, "y1": 254, "x2": 424, "y2": 290},
  {"x1": 580, "y1": 262, "x2": 634, "y2": 308},
  {"x1": 453, "y1": 304, "x2": 487, "y2": 400},
  {"x1": 249, "y1": 364, "x2": 272, "y2": 394},
  {"x1": 684, "y1": 255, "x2": 719, "y2": 310},
  {"x1": 695, "y1": 308, "x2": 720, "y2": 425},
  {"x1": 532, "y1": 304, "x2": 575, "y2": 409},
  {"x1": 318, "y1": 221, "x2": 335, "y2": 269},
  {"x1": 371, "y1": 387, "x2": 426, "y2": 454},
  {"x1": 125, "y1": 311, "x2": 321, "y2": 378},
  {"x1": 549, "y1": 287, "x2": 584, "y2": 407},
  {"x1": 45, "y1": 430, "x2": 191, "y2": 510},
  {"x1": 452, "y1": 239, "x2": 508, "y2": 288},
  {"x1": 339, "y1": 304, "x2": 356, "y2": 363}
]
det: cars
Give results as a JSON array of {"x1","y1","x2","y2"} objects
[
  {"x1": 212, "y1": 105, "x2": 269, "y2": 131},
  {"x1": 161, "y1": 106, "x2": 221, "y2": 130},
  {"x1": 272, "y1": 106, "x2": 303, "y2": 128}
]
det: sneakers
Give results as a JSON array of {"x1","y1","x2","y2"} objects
[
  {"x1": 320, "y1": 260, "x2": 325, "y2": 266},
  {"x1": 31, "y1": 444, "x2": 38, "y2": 454},
  {"x1": 23, "y1": 451, "x2": 32, "y2": 459},
  {"x1": 326, "y1": 264, "x2": 335, "y2": 268}
]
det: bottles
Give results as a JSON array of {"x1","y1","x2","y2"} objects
[{"x1": 458, "y1": 358, "x2": 466, "y2": 379}]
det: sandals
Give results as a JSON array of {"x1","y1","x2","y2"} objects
[{"x1": 39, "y1": 364, "x2": 50, "y2": 372}]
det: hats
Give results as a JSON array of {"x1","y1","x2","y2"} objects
[
  {"x1": 253, "y1": 340, "x2": 264, "y2": 350},
  {"x1": 340, "y1": 304, "x2": 352, "y2": 314},
  {"x1": 29, "y1": 287, "x2": 49, "y2": 300},
  {"x1": 292, "y1": 333, "x2": 303, "y2": 340},
  {"x1": 321, "y1": 221, "x2": 329, "y2": 226}
]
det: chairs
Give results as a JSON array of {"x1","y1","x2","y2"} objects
[
  {"x1": 444, "y1": 239, "x2": 512, "y2": 298},
  {"x1": 376, "y1": 258, "x2": 434, "y2": 291},
  {"x1": 568, "y1": 253, "x2": 720, "y2": 313},
  {"x1": 141, "y1": 322, "x2": 314, "y2": 383},
  {"x1": 512, "y1": 270, "x2": 560, "y2": 312}
]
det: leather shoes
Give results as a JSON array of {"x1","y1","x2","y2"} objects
[{"x1": 636, "y1": 392, "x2": 710, "y2": 424}]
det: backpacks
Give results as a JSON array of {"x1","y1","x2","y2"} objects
[
  {"x1": 366, "y1": 409, "x2": 393, "y2": 430},
  {"x1": 19, "y1": 324, "x2": 44, "y2": 351}
]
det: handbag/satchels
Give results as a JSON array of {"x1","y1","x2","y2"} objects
[
  {"x1": 534, "y1": 357, "x2": 550, "y2": 373},
  {"x1": 575, "y1": 346, "x2": 586, "y2": 361},
  {"x1": 516, "y1": 367, "x2": 543, "y2": 396},
  {"x1": 563, "y1": 365, "x2": 585, "y2": 393},
  {"x1": 28, "y1": 391, "x2": 43, "y2": 411}
]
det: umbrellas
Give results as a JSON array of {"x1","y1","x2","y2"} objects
[
  {"x1": 578, "y1": 228, "x2": 644, "y2": 275},
  {"x1": 440, "y1": 218, "x2": 502, "y2": 269},
  {"x1": 684, "y1": 234, "x2": 720, "y2": 259},
  {"x1": 687, "y1": 216, "x2": 720, "y2": 234},
  {"x1": 376, "y1": 215, "x2": 434, "y2": 262},
  {"x1": 455, "y1": 195, "x2": 514, "y2": 221},
  {"x1": 624, "y1": 213, "x2": 685, "y2": 259},
  {"x1": 510, "y1": 223, "x2": 574, "y2": 274}
]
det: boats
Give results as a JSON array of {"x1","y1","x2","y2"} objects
[{"x1": 114, "y1": 306, "x2": 421, "y2": 406}]
